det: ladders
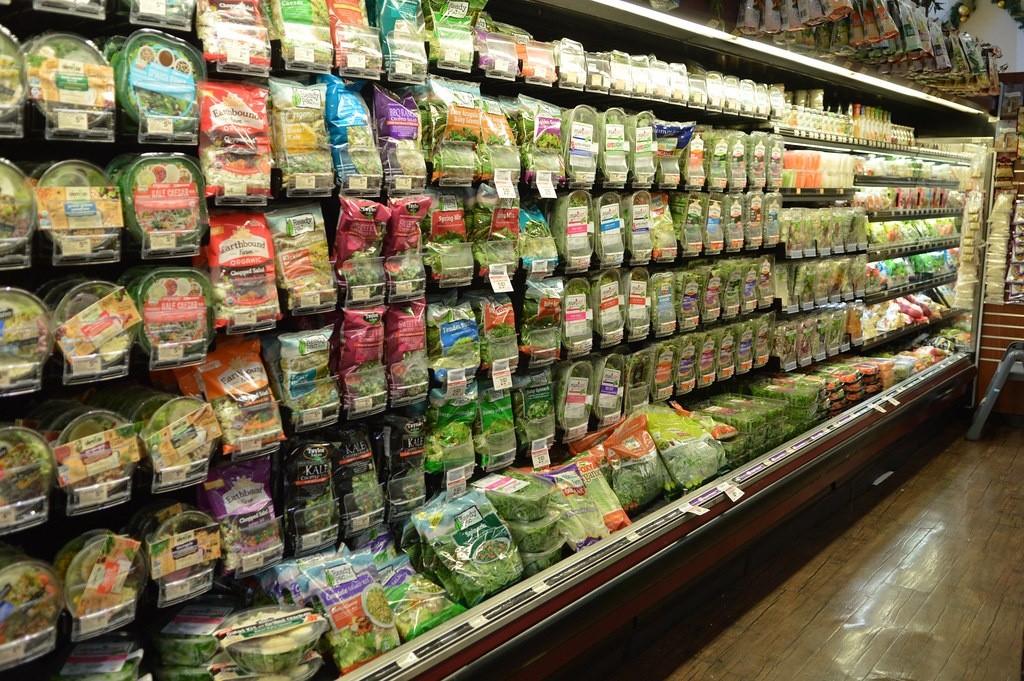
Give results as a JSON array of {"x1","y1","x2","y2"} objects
[{"x1": 963, "y1": 341, "x2": 1024, "y2": 441}]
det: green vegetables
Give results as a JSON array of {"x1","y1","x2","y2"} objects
[{"x1": 0, "y1": 0, "x2": 870, "y2": 681}]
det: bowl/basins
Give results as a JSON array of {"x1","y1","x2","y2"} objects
[
  {"x1": 0, "y1": 22, "x2": 328, "y2": 681},
  {"x1": 551, "y1": 34, "x2": 916, "y2": 495}
]
